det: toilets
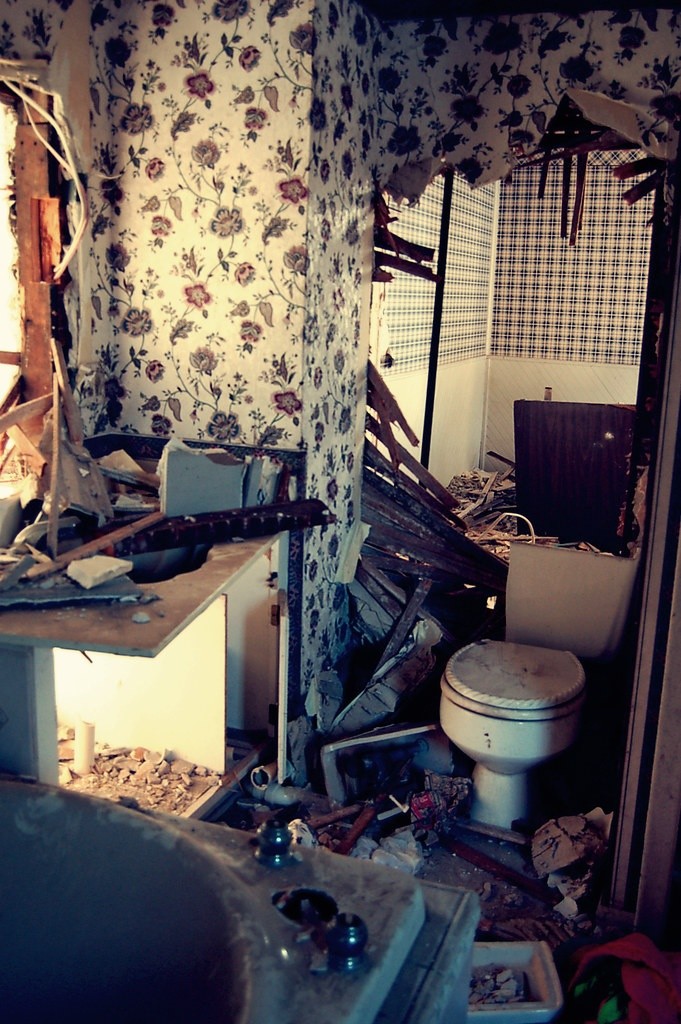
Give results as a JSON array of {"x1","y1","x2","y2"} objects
[{"x1": 440, "y1": 539, "x2": 641, "y2": 830}]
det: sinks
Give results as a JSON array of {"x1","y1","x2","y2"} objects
[{"x1": 0, "y1": 777, "x2": 286, "y2": 1024}]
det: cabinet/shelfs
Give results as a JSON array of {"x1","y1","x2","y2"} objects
[{"x1": 0, "y1": 531, "x2": 288, "y2": 819}]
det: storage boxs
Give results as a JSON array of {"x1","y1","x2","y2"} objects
[{"x1": 465, "y1": 940, "x2": 563, "y2": 1024}]
